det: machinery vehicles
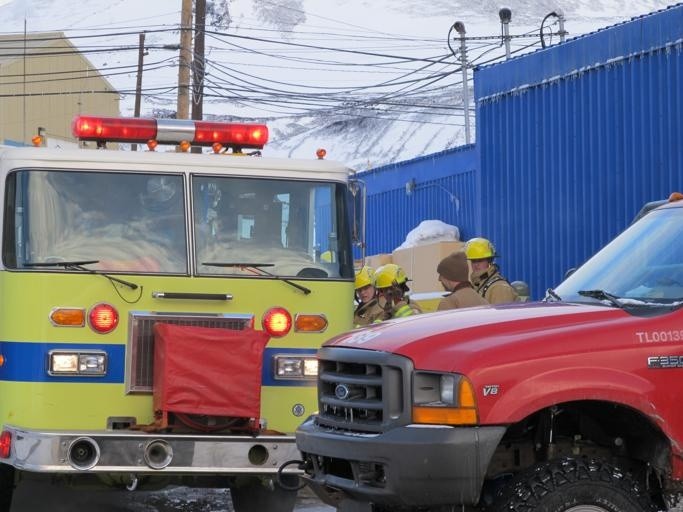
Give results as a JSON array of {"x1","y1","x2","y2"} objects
[{"x1": 0, "y1": 117, "x2": 366, "y2": 508}]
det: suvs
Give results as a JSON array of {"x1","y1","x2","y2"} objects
[{"x1": 295, "y1": 192, "x2": 683, "y2": 508}]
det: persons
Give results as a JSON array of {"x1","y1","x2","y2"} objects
[
  {"x1": 437, "y1": 251, "x2": 490, "y2": 312},
  {"x1": 375, "y1": 264, "x2": 422, "y2": 320},
  {"x1": 464, "y1": 237, "x2": 515, "y2": 305},
  {"x1": 353, "y1": 266, "x2": 388, "y2": 327}
]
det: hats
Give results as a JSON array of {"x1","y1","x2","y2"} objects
[{"x1": 437, "y1": 251, "x2": 469, "y2": 282}]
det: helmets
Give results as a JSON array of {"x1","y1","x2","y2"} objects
[
  {"x1": 321, "y1": 250, "x2": 336, "y2": 265},
  {"x1": 353, "y1": 266, "x2": 375, "y2": 290},
  {"x1": 464, "y1": 237, "x2": 497, "y2": 260},
  {"x1": 375, "y1": 265, "x2": 408, "y2": 288}
]
